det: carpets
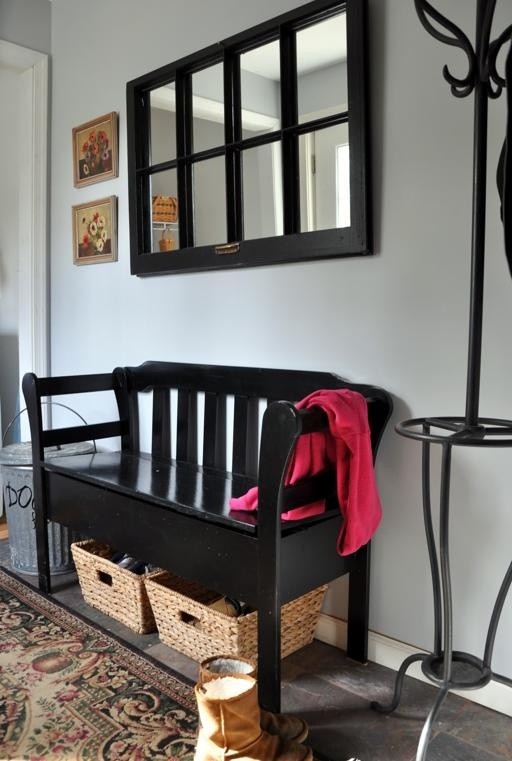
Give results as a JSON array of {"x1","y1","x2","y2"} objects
[{"x1": 0, "y1": 566, "x2": 204, "y2": 761}]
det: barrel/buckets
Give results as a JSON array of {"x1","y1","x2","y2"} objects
[{"x1": 0, "y1": 403, "x2": 95, "y2": 577}]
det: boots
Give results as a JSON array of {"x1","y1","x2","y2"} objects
[{"x1": 195, "y1": 655, "x2": 314, "y2": 761}]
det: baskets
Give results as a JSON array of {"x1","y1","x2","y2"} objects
[
  {"x1": 71, "y1": 539, "x2": 162, "y2": 633},
  {"x1": 143, "y1": 570, "x2": 329, "y2": 666}
]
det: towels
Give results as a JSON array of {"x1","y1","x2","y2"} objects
[{"x1": 229, "y1": 388, "x2": 382, "y2": 557}]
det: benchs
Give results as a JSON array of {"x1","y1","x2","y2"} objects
[{"x1": 22, "y1": 360, "x2": 394, "y2": 713}]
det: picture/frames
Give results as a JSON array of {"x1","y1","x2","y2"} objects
[
  {"x1": 71, "y1": 112, "x2": 118, "y2": 188},
  {"x1": 70, "y1": 195, "x2": 119, "y2": 267}
]
